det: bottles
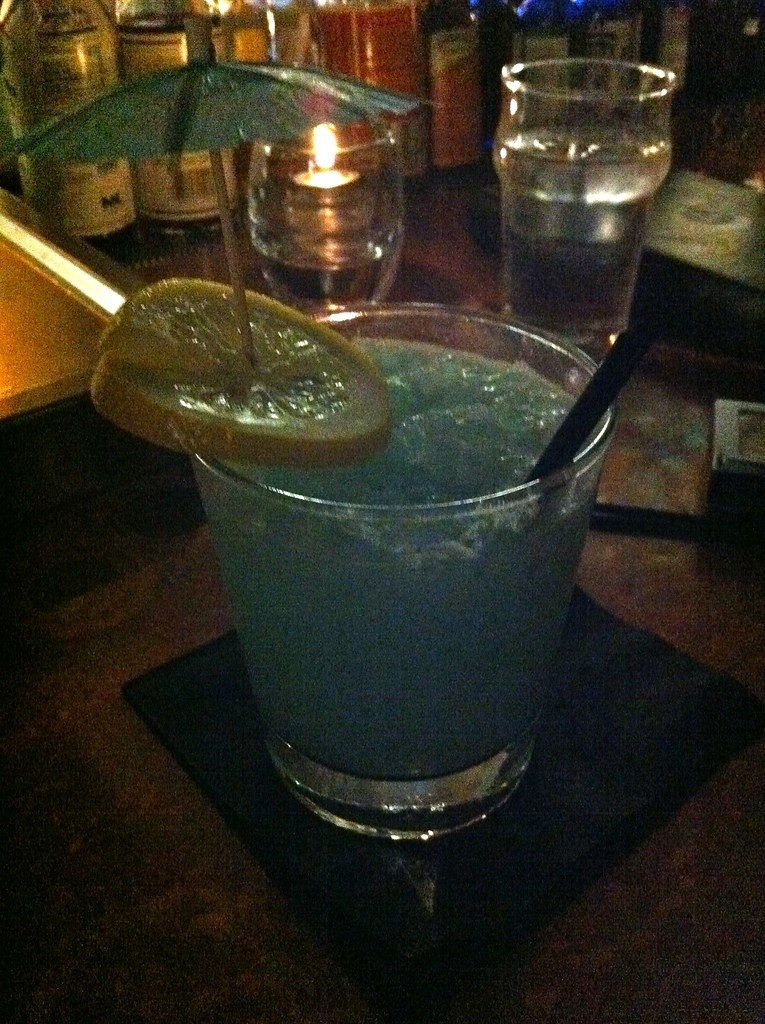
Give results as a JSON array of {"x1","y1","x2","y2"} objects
[{"x1": 0, "y1": 0, "x2": 765, "y2": 253}]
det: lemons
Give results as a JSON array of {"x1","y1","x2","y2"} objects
[{"x1": 91, "y1": 281, "x2": 393, "y2": 468}]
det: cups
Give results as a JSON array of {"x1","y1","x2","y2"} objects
[
  {"x1": 242, "y1": 125, "x2": 409, "y2": 313},
  {"x1": 492, "y1": 57, "x2": 680, "y2": 364},
  {"x1": 193, "y1": 300, "x2": 617, "y2": 839}
]
